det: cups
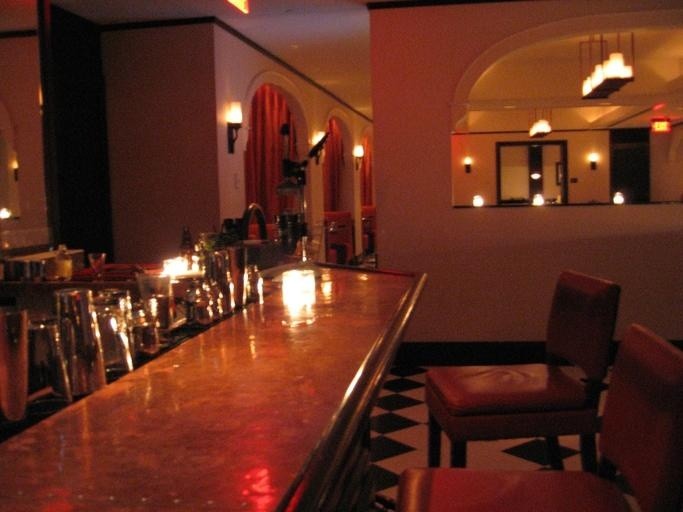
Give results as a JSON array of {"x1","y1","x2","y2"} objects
[
  {"x1": 282, "y1": 269, "x2": 317, "y2": 326},
  {"x1": 86, "y1": 251, "x2": 106, "y2": 279}
]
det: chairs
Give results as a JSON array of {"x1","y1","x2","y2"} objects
[
  {"x1": 424, "y1": 268, "x2": 621, "y2": 473},
  {"x1": 399, "y1": 322, "x2": 683, "y2": 512}
]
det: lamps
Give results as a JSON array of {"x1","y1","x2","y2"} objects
[
  {"x1": 226, "y1": 100, "x2": 243, "y2": 155},
  {"x1": 462, "y1": 156, "x2": 473, "y2": 174},
  {"x1": 588, "y1": 153, "x2": 599, "y2": 170}
]
